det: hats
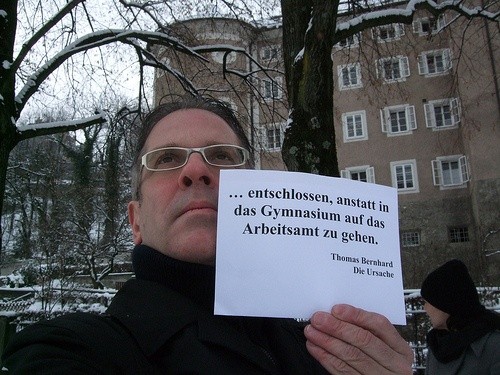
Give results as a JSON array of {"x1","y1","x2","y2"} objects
[{"x1": 420, "y1": 258, "x2": 481, "y2": 316}]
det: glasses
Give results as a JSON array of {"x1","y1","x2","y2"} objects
[{"x1": 137, "y1": 143, "x2": 255, "y2": 186}]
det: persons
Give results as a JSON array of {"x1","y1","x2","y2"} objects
[
  {"x1": 420, "y1": 259, "x2": 500, "y2": 375},
  {"x1": 0, "y1": 100, "x2": 416, "y2": 375}
]
